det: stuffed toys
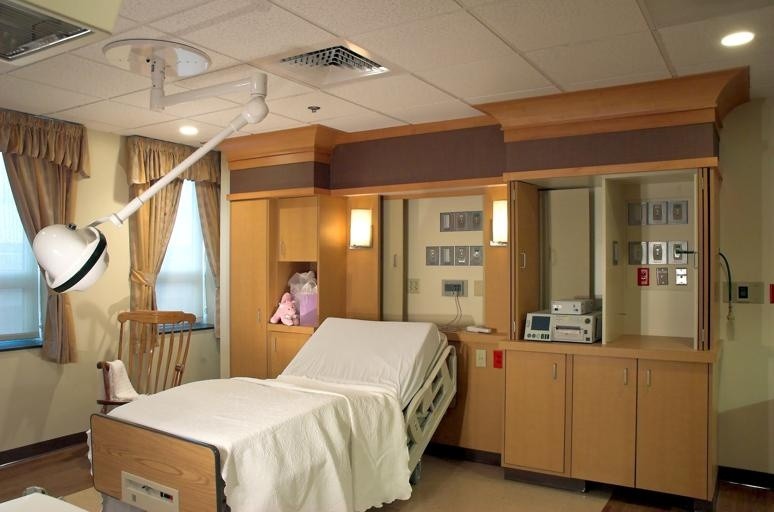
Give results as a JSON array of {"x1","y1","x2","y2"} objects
[{"x1": 270, "y1": 292, "x2": 300, "y2": 326}]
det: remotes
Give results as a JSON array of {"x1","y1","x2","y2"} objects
[{"x1": 467, "y1": 325, "x2": 491, "y2": 334}]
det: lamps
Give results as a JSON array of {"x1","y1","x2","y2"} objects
[
  {"x1": 349, "y1": 207, "x2": 370, "y2": 246},
  {"x1": 31, "y1": 36, "x2": 270, "y2": 296},
  {"x1": 492, "y1": 198, "x2": 507, "y2": 243}
]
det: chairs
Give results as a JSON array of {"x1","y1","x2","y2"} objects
[{"x1": 96, "y1": 310, "x2": 196, "y2": 414}]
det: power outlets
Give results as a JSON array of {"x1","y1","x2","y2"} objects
[
  {"x1": 613, "y1": 198, "x2": 691, "y2": 285},
  {"x1": 477, "y1": 351, "x2": 487, "y2": 369},
  {"x1": 442, "y1": 282, "x2": 463, "y2": 296},
  {"x1": 493, "y1": 351, "x2": 505, "y2": 370}
]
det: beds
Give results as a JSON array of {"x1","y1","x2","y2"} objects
[{"x1": 91, "y1": 316, "x2": 458, "y2": 512}]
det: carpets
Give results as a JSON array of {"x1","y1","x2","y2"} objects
[
  {"x1": 0, "y1": 442, "x2": 94, "y2": 501},
  {"x1": 603, "y1": 485, "x2": 774, "y2": 512}
]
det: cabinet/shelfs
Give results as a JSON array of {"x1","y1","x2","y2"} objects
[
  {"x1": 508, "y1": 182, "x2": 602, "y2": 347},
  {"x1": 276, "y1": 264, "x2": 320, "y2": 326},
  {"x1": 229, "y1": 199, "x2": 271, "y2": 380},
  {"x1": 502, "y1": 348, "x2": 563, "y2": 472},
  {"x1": 604, "y1": 166, "x2": 714, "y2": 349},
  {"x1": 568, "y1": 356, "x2": 708, "y2": 501},
  {"x1": 340, "y1": 194, "x2": 510, "y2": 334},
  {"x1": 268, "y1": 329, "x2": 313, "y2": 379},
  {"x1": 275, "y1": 195, "x2": 317, "y2": 262},
  {"x1": 428, "y1": 342, "x2": 503, "y2": 457}
]
